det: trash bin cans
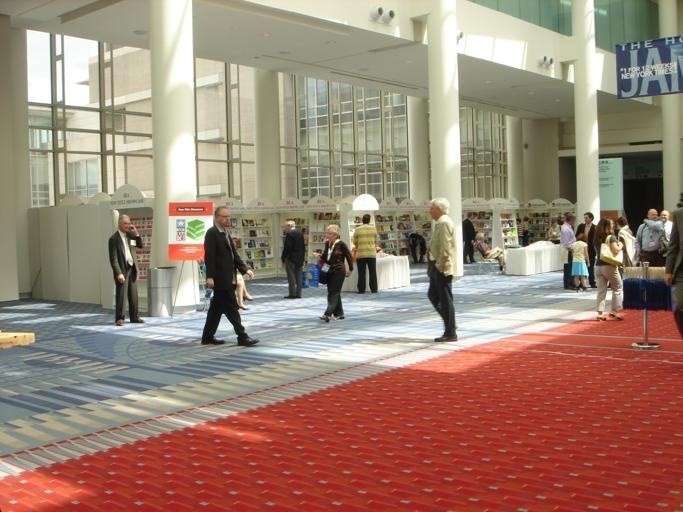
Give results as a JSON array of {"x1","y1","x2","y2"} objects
[{"x1": 146, "y1": 266, "x2": 174, "y2": 317}]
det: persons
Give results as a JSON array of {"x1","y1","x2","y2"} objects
[
  {"x1": 561, "y1": 208, "x2": 674, "y2": 292},
  {"x1": 463, "y1": 213, "x2": 476, "y2": 264},
  {"x1": 201, "y1": 262, "x2": 254, "y2": 310},
  {"x1": 474, "y1": 233, "x2": 506, "y2": 271},
  {"x1": 354, "y1": 214, "x2": 378, "y2": 294},
  {"x1": 664, "y1": 207, "x2": 683, "y2": 337},
  {"x1": 201, "y1": 206, "x2": 259, "y2": 346},
  {"x1": 426, "y1": 196, "x2": 458, "y2": 342},
  {"x1": 109, "y1": 214, "x2": 144, "y2": 326},
  {"x1": 281, "y1": 221, "x2": 305, "y2": 298},
  {"x1": 315, "y1": 224, "x2": 354, "y2": 322},
  {"x1": 592, "y1": 217, "x2": 624, "y2": 321}
]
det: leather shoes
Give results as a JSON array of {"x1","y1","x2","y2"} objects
[
  {"x1": 131, "y1": 318, "x2": 144, "y2": 323},
  {"x1": 116, "y1": 319, "x2": 124, "y2": 326},
  {"x1": 237, "y1": 338, "x2": 259, "y2": 345},
  {"x1": 200, "y1": 339, "x2": 224, "y2": 345}
]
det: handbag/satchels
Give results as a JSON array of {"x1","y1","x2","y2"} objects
[
  {"x1": 599, "y1": 235, "x2": 624, "y2": 266},
  {"x1": 318, "y1": 262, "x2": 331, "y2": 284}
]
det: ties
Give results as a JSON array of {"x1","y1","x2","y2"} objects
[{"x1": 124, "y1": 236, "x2": 133, "y2": 267}]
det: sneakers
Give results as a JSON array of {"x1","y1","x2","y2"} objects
[
  {"x1": 283, "y1": 295, "x2": 301, "y2": 299},
  {"x1": 434, "y1": 335, "x2": 458, "y2": 342},
  {"x1": 609, "y1": 313, "x2": 624, "y2": 321},
  {"x1": 319, "y1": 315, "x2": 330, "y2": 323},
  {"x1": 333, "y1": 314, "x2": 346, "y2": 320},
  {"x1": 576, "y1": 286, "x2": 593, "y2": 294},
  {"x1": 596, "y1": 315, "x2": 607, "y2": 322}
]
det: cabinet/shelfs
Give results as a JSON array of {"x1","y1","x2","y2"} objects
[
  {"x1": 28, "y1": 185, "x2": 154, "y2": 310},
  {"x1": 210, "y1": 195, "x2": 341, "y2": 279},
  {"x1": 342, "y1": 196, "x2": 435, "y2": 265},
  {"x1": 464, "y1": 198, "x2": 574, "y2": 252}
]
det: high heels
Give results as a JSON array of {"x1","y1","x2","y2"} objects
[
  {"x1": 236, "y1": 304, "x2": 250, "y2": 310},
  {"x1": 242, "y1": 296, "x2": 253, "y2": 302}
]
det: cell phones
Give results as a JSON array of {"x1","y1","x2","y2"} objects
[{"x1": 129, "y1": 226, "x2": 133, "y2": 229}]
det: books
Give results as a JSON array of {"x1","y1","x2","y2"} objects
[
  {"x1": 131, "y1": 214, "x2": 153, "y2": 277},
  {"x1": 226, "y1": 213, "x2": 432, "y2": 268},
  {"x1": 471, "y1": 211, "x2": 564, "y2": 251}
]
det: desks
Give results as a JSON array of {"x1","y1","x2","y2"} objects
[{"x1": 623, "y1": 279, "x2": 675, "y2": 311}]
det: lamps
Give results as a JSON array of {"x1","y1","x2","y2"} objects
[
  {"x1": 366, "y1": 5, "x2": 396, "y2": 30},
  {"x1": 534, "y1": 55, "x2": 557, "y2": 68}
]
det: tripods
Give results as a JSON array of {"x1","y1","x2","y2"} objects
[{"x1": 172, "y1": 260, "x2": 208, "y2": 315}]
